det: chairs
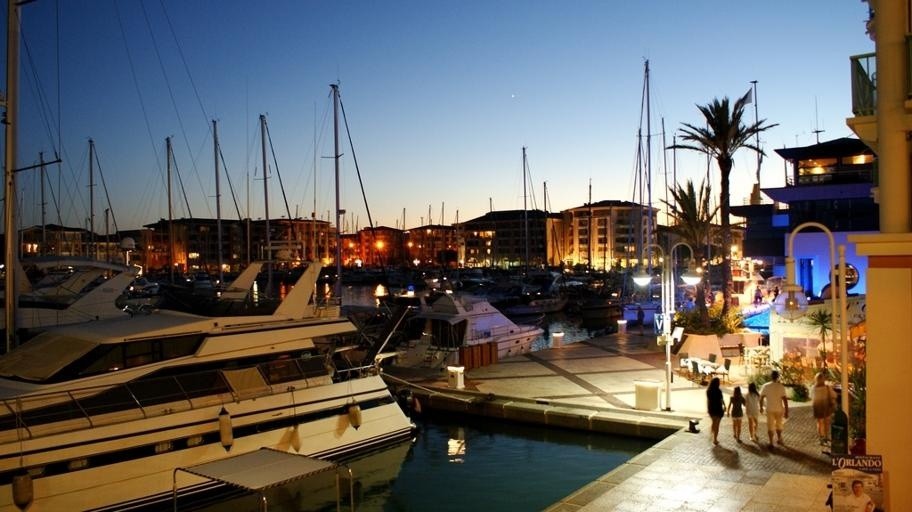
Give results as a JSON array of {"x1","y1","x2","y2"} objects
[{"x1": 674, "y1": 352, "x2": 732, "y2": 388}]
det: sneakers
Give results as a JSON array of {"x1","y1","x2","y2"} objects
[
  {"x1": 712, "y1": 441, "x2": 719, "y2": 444},
  {"x1": 733, "y1": 435, "x2": 742, "y2": 444},
  {"x1": 750, "y1": 433, "x2": 759, "y2": 441}
]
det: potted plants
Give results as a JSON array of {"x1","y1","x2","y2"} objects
[{"x1": 771, "y1": 346, "x2": 803, "y2": 397}]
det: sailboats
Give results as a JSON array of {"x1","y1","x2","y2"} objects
[{"x1": 1, "y1": 0, "x2": 766, "y2": 375}]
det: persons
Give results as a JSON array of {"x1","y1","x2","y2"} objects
[
  {"x1": 726, "y1": 385, "x2": 747, "y2": 443},
  {"x1": 755, "y1": 285, "x2": 763, "y2": 305},
  {"x1": 808, "y1": 372, "x2": 837, "y2": 445},
  {"x1": 772, "y1": 286, "x2": 779, "y2": 301},
  {"x1": 843, "y1": 480, "x2": 876, "y2": 512},
  {"x1": 706, "y1": 376, "x2": 728, "y2": 448},
  {"x1": 637, "y1": 305, "x2": 645, "y2": 335},
  {"x1": 685, "y1": 296, "x2": 694, "y2": 309},
  {"x1": 743, "y1": 383, "x2": 761, "y2": 441},
  {"x1": 757, "y1": 371, "x2": 788, "y2": 447},
  {"x1": 676, "y1": 301, "x2": 683, "y2": 310},
  {"x1": 655, "y1": 305, "x2": 662, "y2": 313}
]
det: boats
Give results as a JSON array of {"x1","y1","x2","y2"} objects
[{"x1": 3, "y1": 260, "x2": 418, "y2": 511}]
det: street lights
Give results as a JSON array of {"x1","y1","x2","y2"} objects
[{"x1": 774, "y1": 222, "x2": 852, "y2": 456}]
